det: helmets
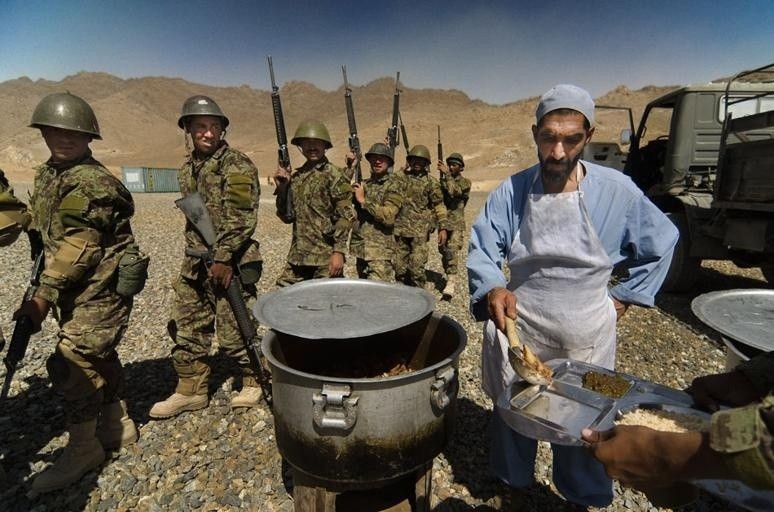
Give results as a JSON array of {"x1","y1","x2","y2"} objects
[
  {"x1": 406, "y1": 145, "x2": 431, "y2": 164},
  {"x1": 27, "y1": 93, "x2": 102, "y2": 139},
  {"x1": 365, "y1": 143, "x2": 394, "y2": 167},
  {"x1": 291, "y1": 120, "x2": 332, "y2": 148},
  {"x1": 178, "y1": 95, "x2": 229, "y2": 129},
  {"x1": 446, "y1": 153, "x2": 464, "y2": 169}
]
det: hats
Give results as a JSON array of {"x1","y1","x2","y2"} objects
[{"x1": 536, "y1": 84, "x2": 594, "y2": 128}]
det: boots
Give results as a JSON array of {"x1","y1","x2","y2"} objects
[
  {"x1": 31, "y1": 399, "x2": 137, "y2": 493},
  {"x1": 148, "y1": 374, "x2": 208, "y2": 418},
  {"x1": 443, "y1": 273, "x2": 455, "y2": 297},
  {"x1": 231, "y1": 362, "x2": 273, "y2": 408}
]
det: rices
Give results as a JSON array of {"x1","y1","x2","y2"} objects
[{"x1": 613, "y1": 408, "x2": 706, "y2": 433}]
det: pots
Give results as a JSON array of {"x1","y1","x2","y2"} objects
[{"x1": 260, "y1": 312, "x2": 468, "y2": 492}]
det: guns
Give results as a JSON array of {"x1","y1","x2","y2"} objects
[
  {"x1": 174, "y1": 190, "x2": 272, "y2": 412},
  {"x1": 437, "y1": 124, "x2": 447, "y2": 184},
  {"x1": 384, "y1": 72, "x2": 403, "y2": 176},
  {"x1": 341, "y1": 65, "x2": 366, "y2": 204},
  {"x1": 0, "y1": 249, "x2": 45, "y2": 413},
  {"x1": 267, "y1": 55, "x2": 296, "y2": 222}
]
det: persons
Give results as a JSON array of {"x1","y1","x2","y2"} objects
[
  {"x1": 272, "y1": 118, "x2": 357, "y2": 292},
  {"x1": 383, "y1": 136, "x2": 448, "y2": 289},
  {"x1": 0, "y1": 168, "x2": 31, "y2": 352},
  {"x1": 580, "y1": 350, "x2": 773, "y2": 510},
  {"x1": 342, "y1": 141, "x2": 404, "y2": 282},
  {"x1": 464, "y1": 83, "x2": 681, "y2": 508},
  {"x1": 147, "y1": 95, "x2": 270, "y2": 421},
  {"x1": 436, "y1": 152, "x2": 472, "y2": 300},
  {"x1": 10, "y1": 92, "x2": 151, "y2": 493}
]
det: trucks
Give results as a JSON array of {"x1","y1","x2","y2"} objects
[{"x1": 580, "y1": 63, "x2": 773, "y2": 296}]
